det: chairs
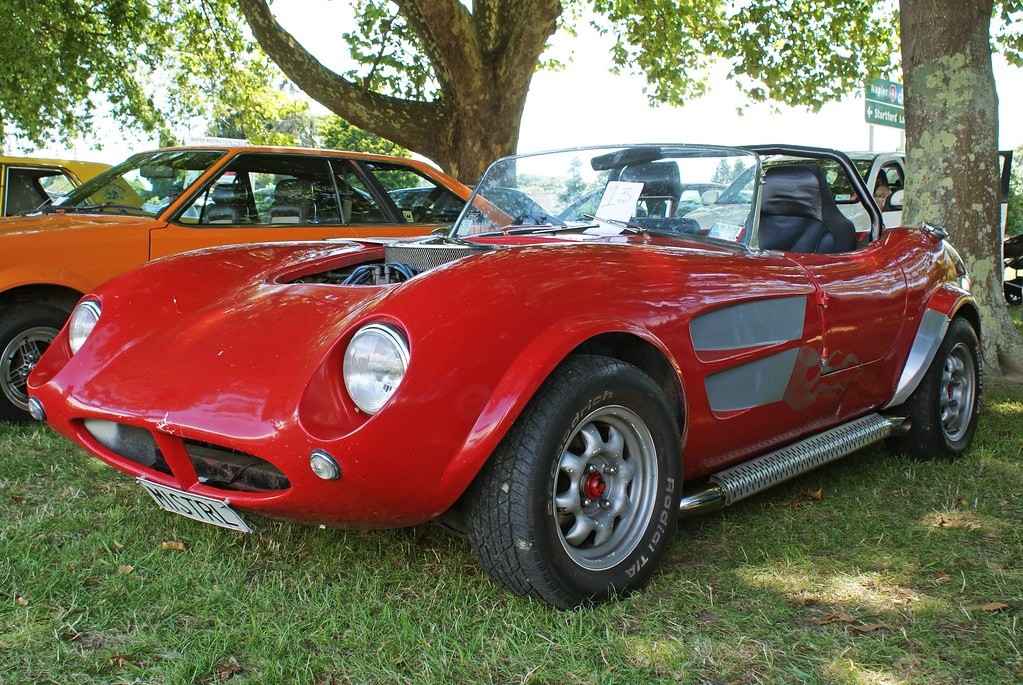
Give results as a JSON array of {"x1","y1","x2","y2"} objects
[
  {"x1": 737, "y1": 162, "x2": 856, "y2": 254},
  {"x1": 594, "y1": 161, "x2": 700, "y2": 235},
  {"x1": 200, "y1": 183, "x2": 249, "y2": 224},
  {"x1": 266, "y1": 178, "x2": 317, "y2": 227}
]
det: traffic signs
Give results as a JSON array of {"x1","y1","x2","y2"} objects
[{"x1": 864, "y1": 99, "x2": 906, "y2": 129}]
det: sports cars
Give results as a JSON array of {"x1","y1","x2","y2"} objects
[{"x1": 25, "y1": 138, "x2": 987, "y2": 612}]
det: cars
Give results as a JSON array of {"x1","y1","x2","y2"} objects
[
  {"x1": 0, "y1": 156, "x2": 147, "y2": 217},
  {"x1": 141, "y1": 181, "x2": 373, "y2": 219},
  {"x1": 685, "y1": 148, "x2": 1014, "y2": 285},
  {"x1": 387, "y1": 187, "x2": 555, "y2": 224},
  {"x1": 0, "y1": 143, "x2": 514, "y2": 427},
  {"x1": 555, "y1": 182, "x2": 754, "y2": 226}
]
